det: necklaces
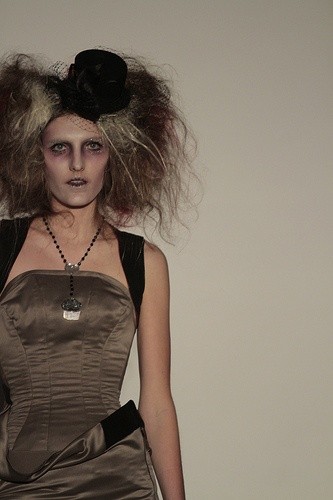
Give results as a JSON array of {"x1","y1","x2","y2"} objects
[{"x1": 42, "y1": 215, "x2": 106, "y2": 321}]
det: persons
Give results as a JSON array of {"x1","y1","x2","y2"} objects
[{"x1": 0, "y1": 47, "x2": 203, "y2": 500}]
